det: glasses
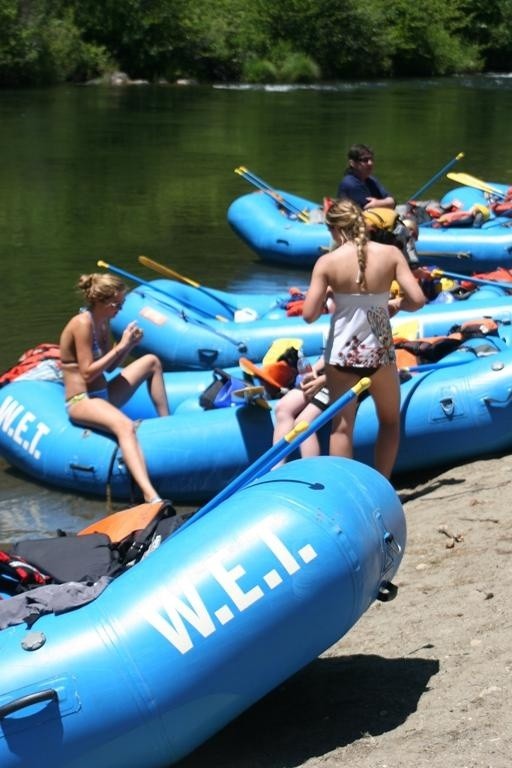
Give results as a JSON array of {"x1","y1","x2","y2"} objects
[
  {"x1": 359, "y1": 157, "x2": 374, "y2": 162},
  {"x1": 106, "y1": 299, "x2": 126, "y2": 308}
]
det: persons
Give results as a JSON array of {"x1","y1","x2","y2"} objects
[
  {"x1": 360, "y1": 207, "x2": 421, "y2": 270},
  {"x1": 270, "y1": 354, "x2": 330, "y2": 472},
  {"x1": 337, "y1": 144, "x2": 397, "y2": 211},
  {"x1": 60, "y1": 273, "x2": 176, "y2": 520},
  {"x1": 302, "y1": 199, "x2": 425, "y2": 479}
]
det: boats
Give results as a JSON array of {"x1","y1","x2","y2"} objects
[
  {"x1": 441, "y1": 183, "x2": 511, "y2": 212},
  {"x1": 0, "y1": 355, "x2": 512, "y2": 499},
  {"x1": 2, "y1": 456, "x2": 406, "y2": 766},
  {"x1": 109, "y1": 279, "x2": 511, "y2": 370},
  {"x1": 227, "y1": 190, "x2": 511, "y2": 273}
]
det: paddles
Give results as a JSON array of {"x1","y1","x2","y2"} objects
[
  {"x1": 262, "y1": 336, "x2": 302, "y2": 370},
  {"x1": 457, "y1": 173, "x2": 506, "y2": 196},
  {"x1": 446, "y1": 172, "x2": 508, "y2": 199},
  {"x1": 234, "y1": 167, "x2": 309, "y2": 223},
  {"x1": 138, "y1": 255, "x2": 240, "y2": 315}
]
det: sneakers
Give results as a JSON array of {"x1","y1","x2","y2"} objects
[{"x1": 157, "y1": 500, "x2": 177, "y2": 518}]
